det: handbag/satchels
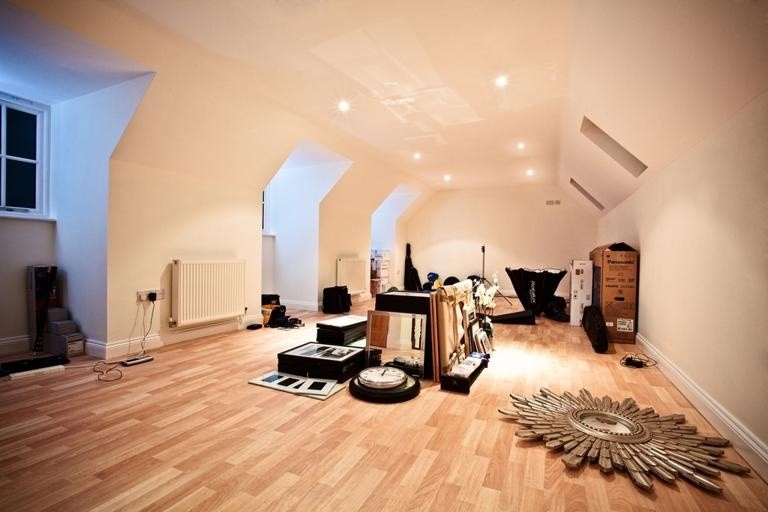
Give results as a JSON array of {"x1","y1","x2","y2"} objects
[{"x1": 264, "y1": 305, "x2": 305, "y2": 329}]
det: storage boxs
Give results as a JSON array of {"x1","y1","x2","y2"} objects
[
  {"x1": 569, "y1": 240, "x2": 640, "y2": 344},
  {"x1": 27, "y1": 265, "x2": 85, "y2": 358}
]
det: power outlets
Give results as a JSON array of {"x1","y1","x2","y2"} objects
[{"x1": 137, "y1": 288, "x2": 165, "y2": 301}]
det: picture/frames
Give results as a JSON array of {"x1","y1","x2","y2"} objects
[
  {"x1": 430, "y1": 277, "x2": 492, "y2": 383},
  {"x1": 277, "y1": 340, "x2": 366, "y2": 384}
]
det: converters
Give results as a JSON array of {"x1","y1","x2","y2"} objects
[{"x1": 149, "y1": 293, "x2": 157, "y2": 300}]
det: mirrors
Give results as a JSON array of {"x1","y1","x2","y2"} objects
[{"x1": 367, "y1": 311, "x2": 426, "y2": 382}]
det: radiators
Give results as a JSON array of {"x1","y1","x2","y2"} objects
[
  {"x1": 168, "y1": 258, "x2": 248, "y2": 328},
  {"x1": 336, "y1": 256, "x2": 367, "y2": 297}
]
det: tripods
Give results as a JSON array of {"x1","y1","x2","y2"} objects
[{"x1": 473, "y1": 252, "x2": 512, "y2": 307}]
